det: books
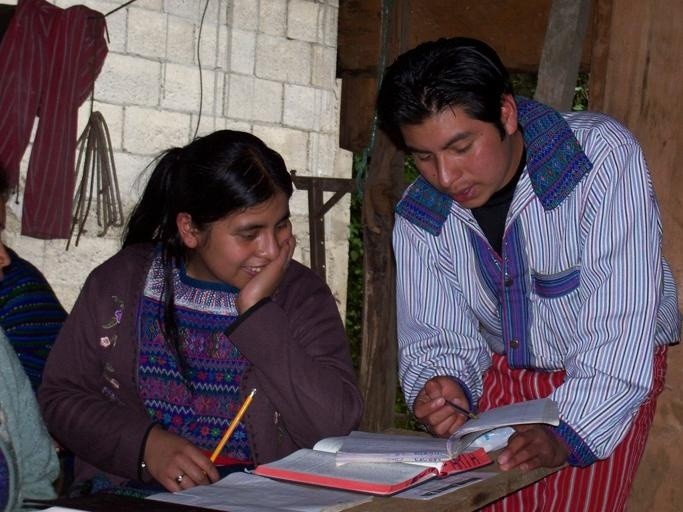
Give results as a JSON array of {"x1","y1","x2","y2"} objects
[
  {"x1": 333, "y1": 397, "x2": 560, "y2": 464},
  {"x1": 254, "y1": 431, "x2": 494, "y2": 497}
]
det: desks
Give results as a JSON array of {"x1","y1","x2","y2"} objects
[{"x1": 57, "y1": 427, "x2": 564, "y2": 510}]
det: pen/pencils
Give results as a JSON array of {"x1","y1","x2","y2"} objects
[
  {"x1": 444, "y1": 399, "x2": 479, "y2": 421},
  {"x1": 201, "y1": 387, "x2": 257, "y2": 475}
]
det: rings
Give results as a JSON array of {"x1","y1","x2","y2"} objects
[{"x1": 175, "y1": 474, "x2": 184, "y2": 483}]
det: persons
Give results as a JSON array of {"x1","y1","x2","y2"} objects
[
  {"x1": 370, "y1": 34, "x2": 683, "y2": 512},
  {"x1": 0, "y1": 162, "x2": 68, "y2": 510},
  {"x1": 28, "y1": 127, "x2": 366, "y2": 495}
]
